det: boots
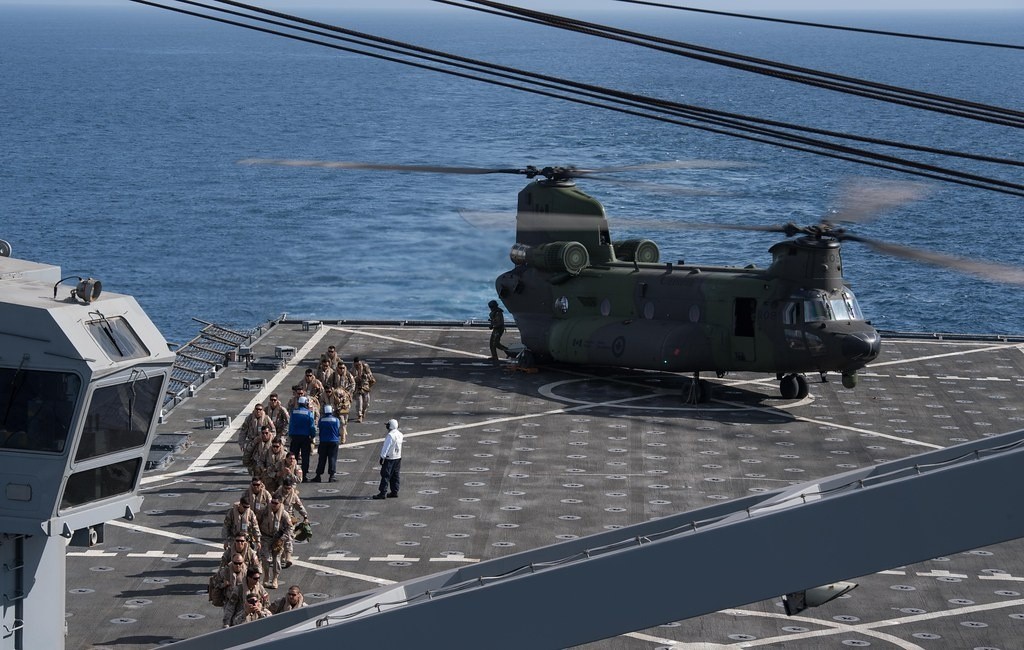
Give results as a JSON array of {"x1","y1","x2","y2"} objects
[
  {"x1": 272, "y1": 572, "x2": 279, "y2": 589},
  {"x1": 263, "y1": 575, "x2": 271, "y2": 587}
]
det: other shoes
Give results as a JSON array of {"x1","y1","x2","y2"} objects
[
  {"x1": 329, "y1": 476, "x2": 340, "y2": 482},
  {"x1": 283, "y1": 562, "x2": 292, "y2": 569},
  {"x1": 372, "y1": 492, "x2": 386, "y2": 499},
  {"x1": 302, "y1": 476, "x2": 308, "y2": 483},
  {"x1": 311, "y1": 476, "x2": 321, "y2": 482},
  {"x1": 341, "y1": 438, "x2": 346, "y2": 443},
  {"x1": 387, "y1": 492, "x2": 398, "y2": 499}
]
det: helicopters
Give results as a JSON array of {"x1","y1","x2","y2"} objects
[{"x1": 236, "y1": 157, "x2": 1024, "y2": 402}]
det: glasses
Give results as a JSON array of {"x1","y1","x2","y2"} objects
[
  {"x1": 242, "y1": 504, "x2": 249, "y2": 508},
  {"x1": 272, "y1": 445, "x2": 279, "y2": 448},
  {"x1": 250, "y1": 577, "x2": 260, "y2": 581},
  {"x1": 262, "y1": 432, "x2": 268, "y2": 435},
  {"x1": 236, "y1": 540, "x2": 245, "y2": 542},
  {"x1": 252, "y1": 483, "x2": 260, "y2": 486},
  {"x1": 232, "y1": 560, "x2": 244, "y2": 565},
  {"x1": 297, "y1": 350, "x2": 361, "y2": 395},
  {"x1": 284, "y1": 487, "x2": 291, "y2": 490},
  {"x1": 256, "y1": 408, "x2": 262, "y2": 411},
  {"x1": 270, "y1": 399, "x2": 277, "y2": 401},
  {"x1": 271, "y1": 501, "x2": 279, "y2": 505},
  {"x1": 288, "y1": 593, "x2": 296, "y2": 596}
]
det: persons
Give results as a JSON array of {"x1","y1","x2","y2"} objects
[
  {"x1": 207, "y1": 393, "x2": 312, "y2": 628},
  {"x1": 487, "y1": 300, "x2": 510, "y2": 360},
  {"x1": 287, "y1": 346, "x2": 356, "y2": 459},
  {"x1": 289, "y1": 397, "x2": 317, "y2": 484},
  {"x1": 373, "y1": 419, "x2": 403, "y2": 499},
  {"x1": 351, "y1": 357, "x2": 376, "y2": 423},
  {"x1": 312, "y1": 406, "x2": 340, "y2": 483}
]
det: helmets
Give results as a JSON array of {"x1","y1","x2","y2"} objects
[
  {"x1": 324, "y1": 405, "x2": 332, "y2": 414},
  {"x1": 298, "y1": 397, "x2": 309, "y2": 404},
  {"x1": 384, "y1": 419, "x2": 398, "y2": 430}
]
